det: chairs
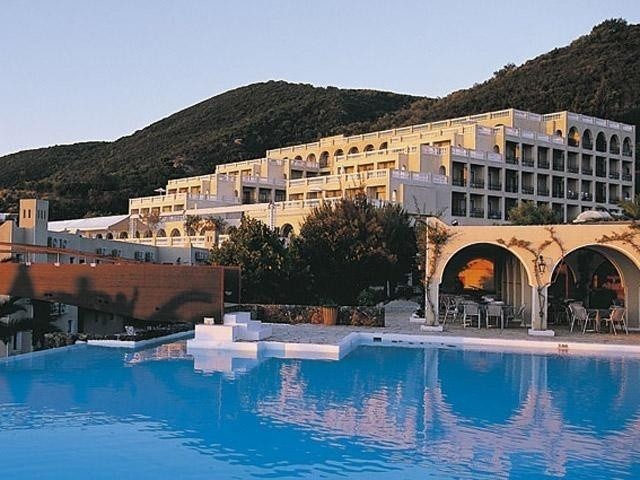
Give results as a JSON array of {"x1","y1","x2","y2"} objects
[
  {"x1": 438, "y1": 293, "x2": 526, "y2": 334},
  {"x1": 548, "y1": 299, "x2": 627, "y2": 334}
]
